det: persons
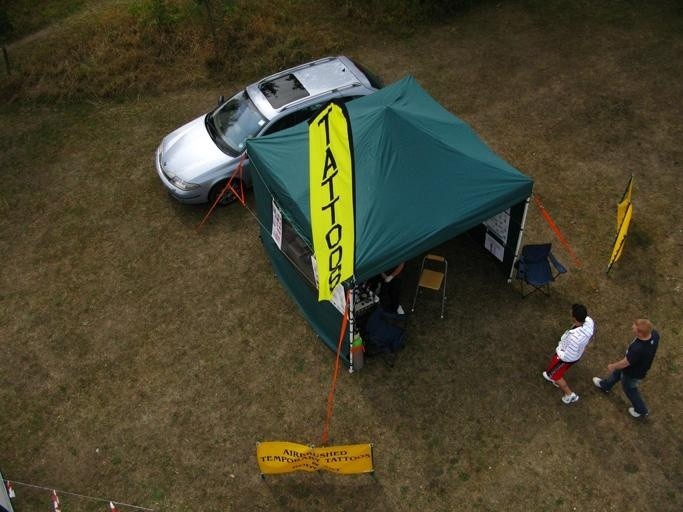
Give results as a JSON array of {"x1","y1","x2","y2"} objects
[
  {"x1": 375, "y1": 261, "x2": 410, "y2": 314},
  {"x1": 542, "y1": 302, "x2": 596, "y2": 405},
  {"x1": 592, "y1": 317, "x2": 661, "y2": 418}
]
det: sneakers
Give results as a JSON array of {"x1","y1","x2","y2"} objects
[
  {"x1": 542, "y1": 371, "x2": 559, "y2": 387},
  {"x1": 562, "y1": 393, "x2": 579, "y2": 404},
  {"x1": 593, "y1": 377, "x2": 603, "y2": 388},
  {"x1": 628, "y1": 407, "x2": 649, "y2": 418}
]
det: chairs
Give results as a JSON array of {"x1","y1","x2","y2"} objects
[
  {"x1": 515, "y1": 242, "x2": 567, "y2": 299},
  {"x1": 409, "y1": 255, "x2": 449, "y2": 319}
]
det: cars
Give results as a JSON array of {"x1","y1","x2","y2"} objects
[{"x1": 155, "y1": 54, "x2": 387, "y2": 207}]
